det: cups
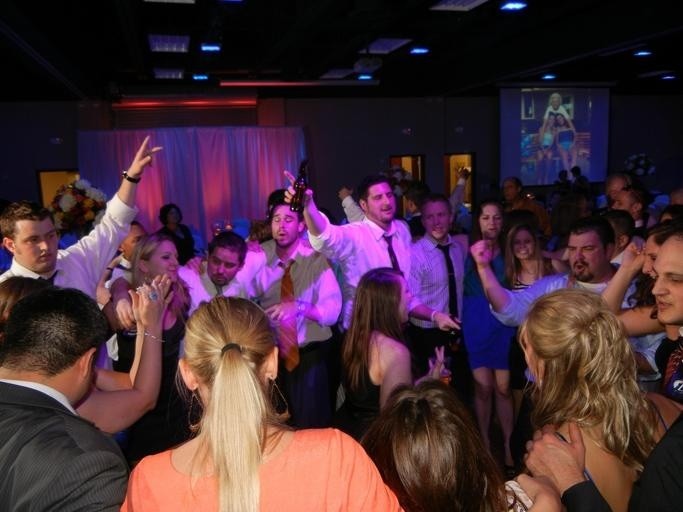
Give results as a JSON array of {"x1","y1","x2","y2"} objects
[{"x1": 430, "y1": 357, "x2": 450, "y2": 388}]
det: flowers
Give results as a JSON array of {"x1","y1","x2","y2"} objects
[
  {"x1": 48, "y1": 178, "x2": 108, "y2": 238},
  {"x1": 623, "y1": 152, "x2": 657, "y2": 176}
]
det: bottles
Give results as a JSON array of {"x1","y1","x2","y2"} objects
[{"x1": 288, "y1": 160, "x2": 310, "y2": 213}]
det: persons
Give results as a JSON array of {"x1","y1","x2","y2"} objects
[
  {"x1": 533, "y1": 93, "x2": 582, "y2": 185},
  {"x1": 2, "y1": 136, "x2": 682, "y2": 512}
]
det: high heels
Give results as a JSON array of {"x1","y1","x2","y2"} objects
[{"x1": 503, "y1": 456, "x2": 516, "y2": 478}]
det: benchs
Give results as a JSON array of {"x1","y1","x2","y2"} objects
[{"x1": 520, "y1": 132, "x2": 592, "y2": 187}]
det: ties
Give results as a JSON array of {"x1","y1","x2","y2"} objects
[
  {"x1": 216, "y1": 285, "x2": 223, "y2": 298},
  {"x1": 384, "y1": 236, "x2": 404, "y2": 276},
  {"x1": 279, "y1": 259, "x2": 300, "y2": 374},
  {"x1": 436, "y1": 244, "x2": 460, "y2": 320}
]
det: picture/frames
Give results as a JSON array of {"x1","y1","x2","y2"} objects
[
  {"x1": 520, "y1": 94, "x2": 534, "y2": 120},
  {"x1": 562, "y1": 95, "x2": 575, "y2": 119}
]
